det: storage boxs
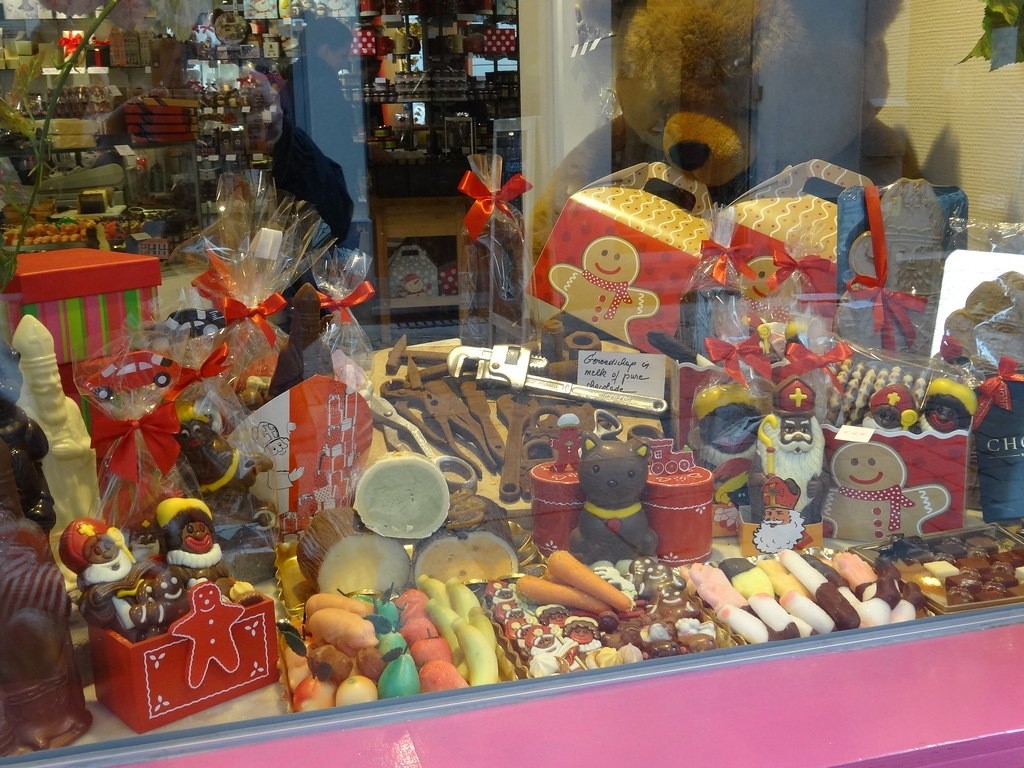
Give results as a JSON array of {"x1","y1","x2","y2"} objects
[
  {"x1": 523, "y1": 159, "x2": 1024, "y2": 614},
  {"x1": 388, "y1": 246, "x2": 439, "y2": 298},
  {"x1": 0, "y1": 24, "x2": 200, "y2": 145},
  {"x1": 0, "y1": 247, "x2": 162, "y2": 438},
  {"x1": 86, "y1": 575, "x2": 282, "y2": 733}
]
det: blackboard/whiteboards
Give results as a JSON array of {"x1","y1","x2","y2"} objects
[{"x1": 487, "y1": 117, "x2": 536, "y2": 338}]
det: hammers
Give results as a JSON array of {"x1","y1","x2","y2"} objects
[
  {"x1": 385, "y1": 334, "x2": 449, "y2": 374},
  {"x1": 405, "y1": 354, "x2": 449, "y2": 390}
]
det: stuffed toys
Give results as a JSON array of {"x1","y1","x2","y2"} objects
[{"x1": 527, "y1": 0, "x2": 922, "y2": 336}]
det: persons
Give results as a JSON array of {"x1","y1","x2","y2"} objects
[{"x1": 274, "y1": 10, "x2": 396, "y2": 249}]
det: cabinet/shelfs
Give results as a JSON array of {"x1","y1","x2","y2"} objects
[
  {"x1": 188, "y1": 54, "x2": 300, "y2": 227},
  {"x1": 0, "y1": 141, "x2": 205, "y2": 272},
  {"x1": 355, "y1": 14, "x2": 521, "y2": 198}
]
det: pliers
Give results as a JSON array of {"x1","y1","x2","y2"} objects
[
  {"x1": 420, "y1": 389, "x2": 498, "y2": 480},
  {"x1": 369, "y1": 394, "x2": 434, "y2": 463},
  {"x1": 380, "y1": 378, "x2": 448, "y2": 446}
]
